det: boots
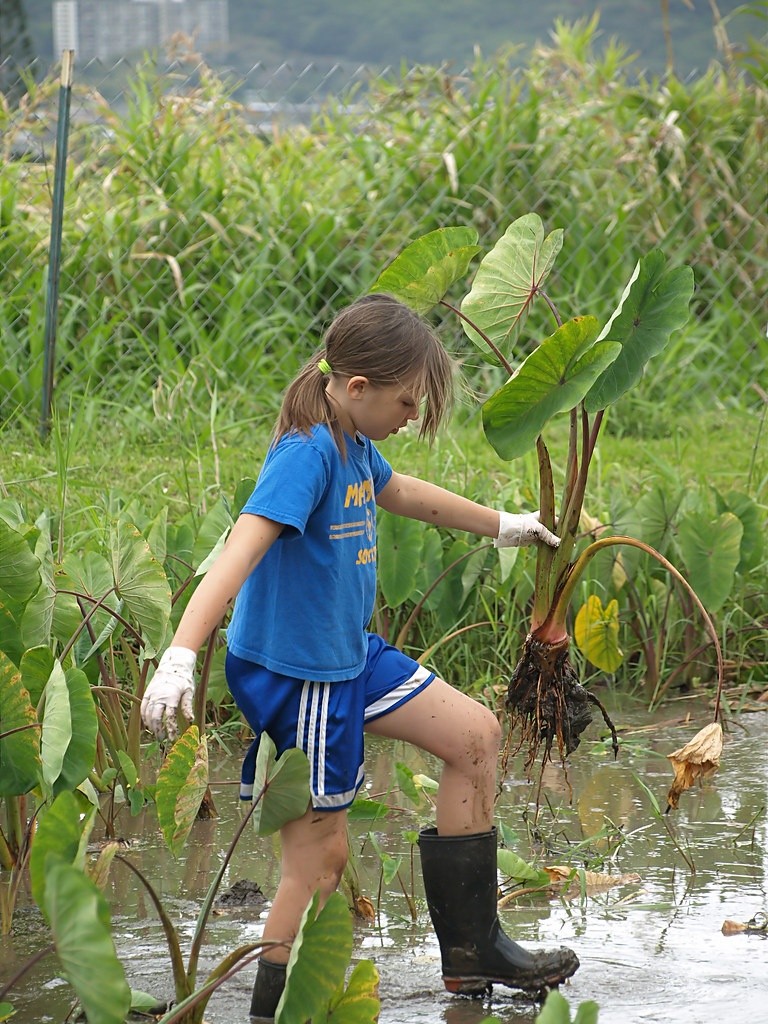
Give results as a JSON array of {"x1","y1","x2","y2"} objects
[
  {"x1": 416, "y1": 826, "x2": 580, "y2": 992},
  {"x1": 249, "y1": 958, "x2": 288, "y2": 1020}
]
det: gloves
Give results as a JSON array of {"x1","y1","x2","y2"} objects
[
  {"x1": 494, "y1": 511, "x2": 577, "y2": 548},
  {"x1": 140, "y1": 647, "x2": 197, "y2": 743}
]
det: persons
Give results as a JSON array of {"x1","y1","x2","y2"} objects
[{"x1": 139, "y1": 294, "x2": 581, "y2": 1018}]
